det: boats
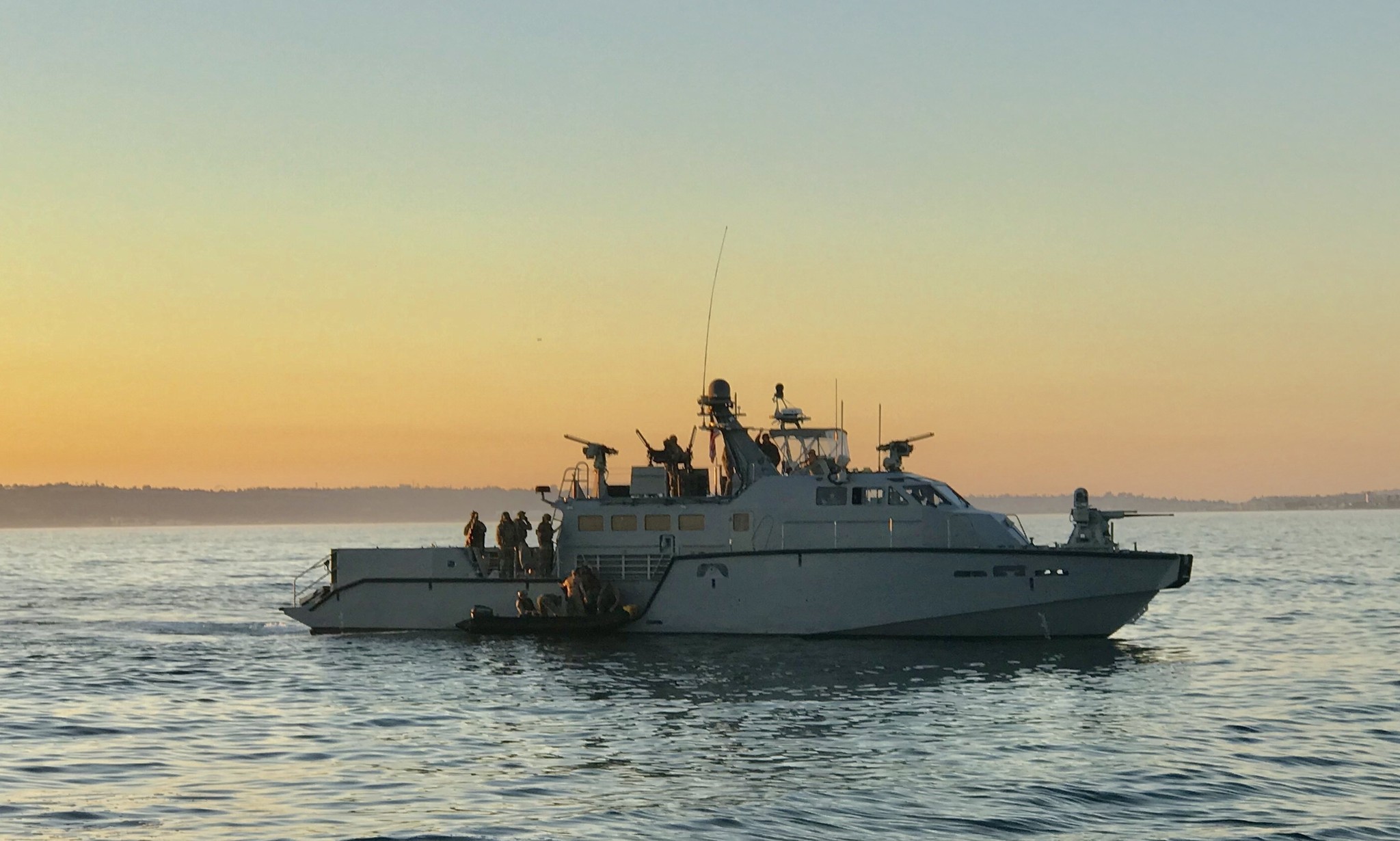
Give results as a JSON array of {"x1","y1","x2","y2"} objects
[
  {"x1": 456, "y1": 605, "x2": 614, "y2": 638},
  {"x1": 278, "y1": 224, "x2": 1196, "y2": 633}
]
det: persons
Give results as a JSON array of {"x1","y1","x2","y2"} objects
[
  {"x1": 497, "y1": 510, "x2": 558, "y2": 579},
  {"x1": 464, "y1": 510, "x2": 490, "y2": 580},
  {"x1": 754, "y1": 427, "x2": 780, "y2": 468},
  {"x1": 536, "y1": 593, "x2": 564, "y2": 617},
  {"x1": 516, "y1": 589, "x2": 536, "y2": 618},
  {"x1": 652, "y1": 435, "x2": 694, "y2": 499},
  {"x1": 557, "y1": 569, "x2": 620, "y2": 618}
]
